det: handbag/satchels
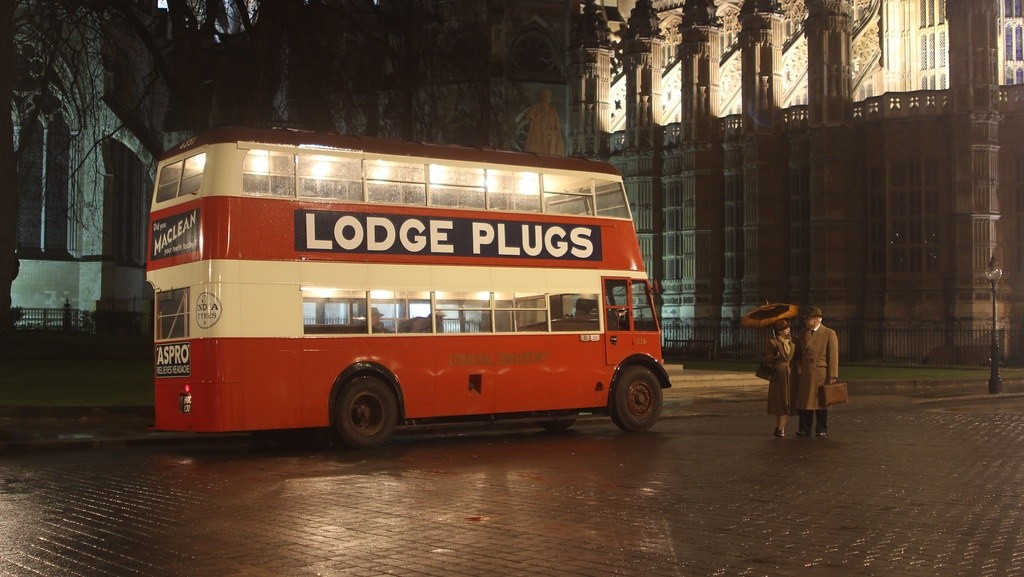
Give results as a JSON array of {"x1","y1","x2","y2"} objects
[{"x1": 755, "y1": 364, "x2": 774, "y2": 380}]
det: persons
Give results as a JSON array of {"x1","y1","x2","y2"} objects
[
  {"x1": 767, "y1": 307, "x2": 838, "y2": 439},
  {"x1": 363, "y1": 307, "x2": 388, "y2": 334},
  {"x1": 576, "y1": 294, "x2": 625, "y2": 331}
]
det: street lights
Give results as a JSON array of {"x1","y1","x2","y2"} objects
[{"x1": 986, "y1": 253, "x2": 1003, "y2": 394}]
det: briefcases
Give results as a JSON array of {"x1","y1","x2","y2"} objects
[{"x1": 818, "y1": 381, "x2": 848, "y2": 406}]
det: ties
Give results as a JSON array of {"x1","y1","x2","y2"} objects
[{"x1": 811, "y1": 330, "x2": 815, "y2": 334}]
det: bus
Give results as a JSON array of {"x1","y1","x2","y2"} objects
[{"x1": 146, "y1": 123, "x2": 672, "y2": 451}]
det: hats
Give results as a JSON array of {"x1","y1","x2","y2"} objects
[
  {"x1": 775, "y1": 319, "x2": 790, "y2": 331},
  {"x1": 800, "y1": 307, "x2": 824, "y2": 319}
]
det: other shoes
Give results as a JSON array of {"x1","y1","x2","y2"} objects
[
  {"x1": 775, "y1": 427, "x2": 785, "y2": 438},
  {"x1": 816, "y1": 432, "x2": 832, "y2": 439},
  {"x1": 797, "y1": 432, "x2": 810, "y2": 437}
]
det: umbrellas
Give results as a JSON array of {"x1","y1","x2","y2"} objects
[{"x1": 741, "y1": 300, "x2": 798, "y2": 340}]
